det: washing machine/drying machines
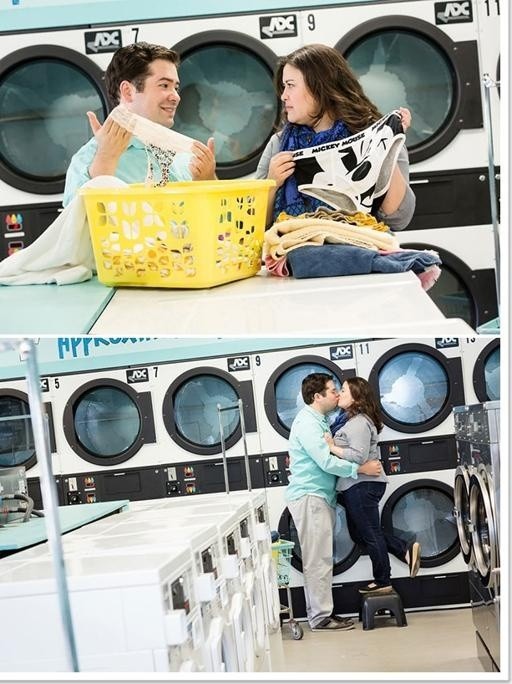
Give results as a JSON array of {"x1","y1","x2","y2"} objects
[{"x1": 0, "y1": 0, "x2": 501, "y2": 673}]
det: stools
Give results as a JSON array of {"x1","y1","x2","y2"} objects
[{"x1": 358, "y1": 590, "x2": 407, "y2": 630}]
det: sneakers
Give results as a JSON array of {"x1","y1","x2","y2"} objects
[
  {"x1": 328, "y1": 614, "x2": 354, "y2": 626},
  {"x1": 311, "y1": 616, "x2": 357, "y2": 633},
  {"x1": 406, "y1": 542, "x2": 423, "y2": 580},
  {"x1": 358, "y1": 581, "x2": 394, "y2": 595}
]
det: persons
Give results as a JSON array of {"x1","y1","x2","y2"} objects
[
  {"x1": 323, "y1": 377, "x2": 421, "y2": 593},
  {"x1": 252, "y1": 41, "x2": 415, "y2": 231},
  {"x1": 285, "y1": 371, "x2": 381, "y2": 631},
  {"x1": 65, "y1": 42, "x2": 215, "y2": 274}
]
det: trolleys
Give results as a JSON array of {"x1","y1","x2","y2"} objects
[{"x1": 274, "y1": 538, "x2": 302, "y2": 644}]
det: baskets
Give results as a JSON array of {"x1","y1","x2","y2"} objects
[{"x1": 76, "y1": 174, "x2": 278, "y2": 291}]
